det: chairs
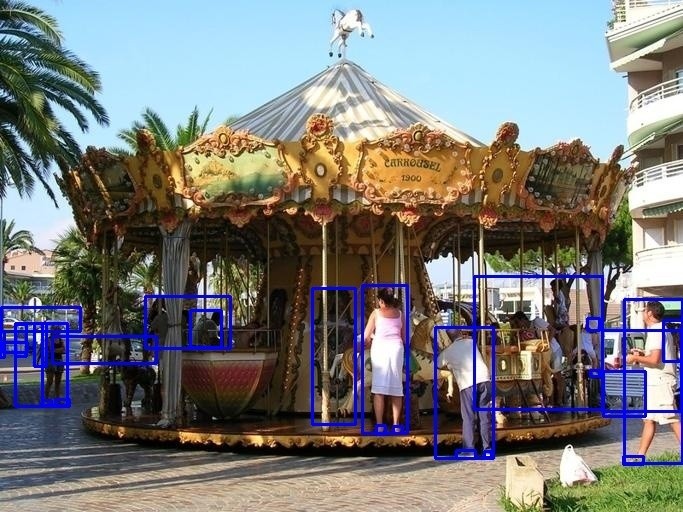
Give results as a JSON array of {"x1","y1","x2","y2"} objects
[
  {"x1": 591, "y1": 371, "x2": 599, "y2": 377},
  {"x1": 484, "y1": 452, "x2": 492, "y2": 458},
  {"x1": 457, "y1": 451, "x2": 475, "y2": 458}
]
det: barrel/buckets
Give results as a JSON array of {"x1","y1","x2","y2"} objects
[
  {"x1": 377, "y1": 426, "x2": 386, "y2": 435},
  {"x1": 393, "y1": 427, "x2": 403, "y2": 433}
]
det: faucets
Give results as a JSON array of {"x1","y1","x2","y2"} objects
[
  {"x1": 56, "y1": 400, "x2": 69, "y2": 406},
  {"x1": 623, "y1": 456, "x2": 644, "y2": 465},
  {"x1": 43, "y1": 400, "x2": 52, "y2": 406}
]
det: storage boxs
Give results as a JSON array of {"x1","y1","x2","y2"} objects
[{"x1": 603, "y1": 371, "x2": 645, "y2": 416}]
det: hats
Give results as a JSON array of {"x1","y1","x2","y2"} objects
[
  {"x1": 52, "y1": 337, "x2": 157, "y2": 364},
  {"x1": 1, "y1": 307, "x2": 80, "y2": 332},
  {"x1": 602, "y1": 330, "x2": 682, "y2": 411}
]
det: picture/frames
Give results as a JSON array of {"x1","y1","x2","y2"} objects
[{"x1": 4, "y1": 343, "x2": 27, "y2": 353}]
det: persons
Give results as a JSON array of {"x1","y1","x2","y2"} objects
[
  {"x1": 363, "y1": 286, "x2": 407, "y2": 433},
  {"x1": 475, "y1": 277, "x2": 603, "y2": 410},
  {"x1": 15, "y1": 324, "x2": 67, "y2": 407},
  {"x1": 0, "y1": 331, "x2": 5, "y2": 358},
  {"x1": 435, "y1": 328, "x2": 493, "y2": 459},
  {"x1": 146, "y1": 296, "x2": 231, "y2": 348},
  {"x1": 663, "y1": 321, "x2": 683, "y2": 362},
  {"x1": 625, "y1": 299, "x2": 683, "y2": 464}
]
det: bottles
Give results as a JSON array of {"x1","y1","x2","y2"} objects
[{"x1": 588, "y1": 318, "x2": 600, "y2": 331}]
